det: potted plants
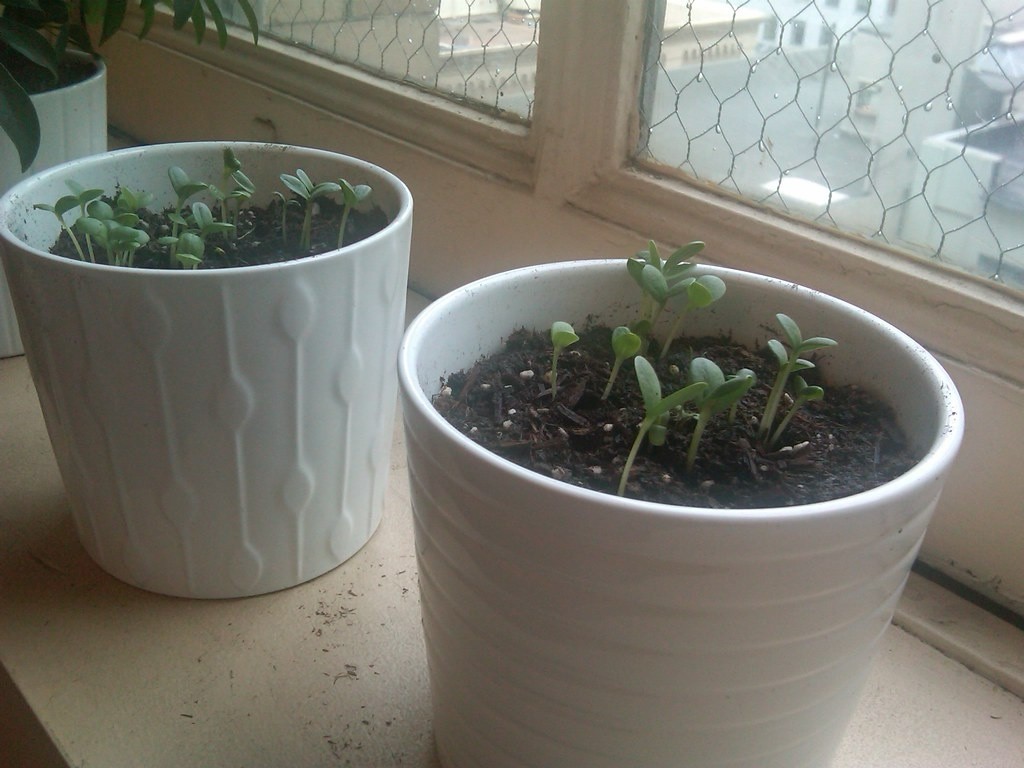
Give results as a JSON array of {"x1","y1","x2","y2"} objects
[
  {"x1": 0, "y1": 0, "x2": 260, "y2": 359},
  {"x1": 0, "y1": 141, "x2": 412, "y2": 599},
  {"x1": 399, "y1": 240, "x2": 965, "y2": 768}
]
